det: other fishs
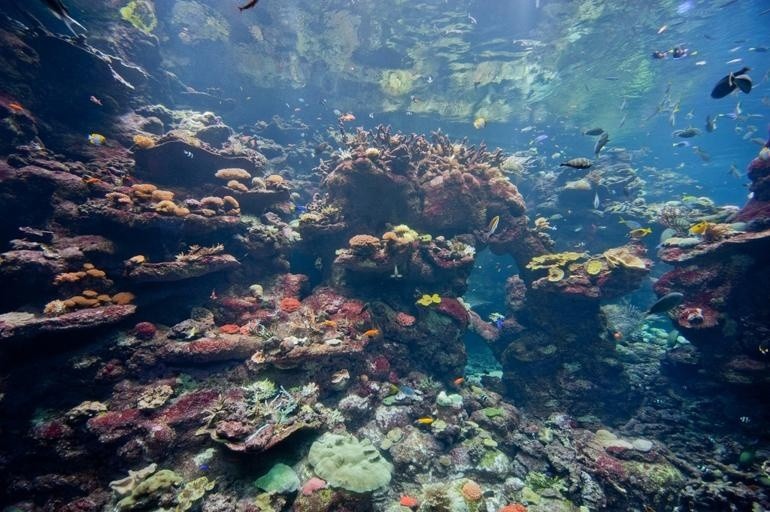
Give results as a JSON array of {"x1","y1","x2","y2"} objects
[{"x1": 210, "y1": 0, "x2": 770, "y2": 406}]
